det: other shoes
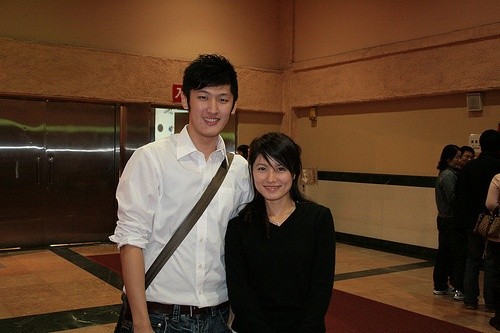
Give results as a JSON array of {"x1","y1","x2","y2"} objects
[
  {"x1": 485, "y1": 306, "x2": 496, "y2": 312},
  {"x1": 490, "y1": 317, "x2": 500, "y2": 330},
  {"x1": 466, "y1": 303, "x2": 474, "y2": 309}
]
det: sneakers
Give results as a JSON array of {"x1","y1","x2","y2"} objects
[
  {"x1": 454, "y1": 290, "x2": 464, "y2": 300},
  {"x1": 433, "y1": 286, "x2": 457, "y2": 295}
]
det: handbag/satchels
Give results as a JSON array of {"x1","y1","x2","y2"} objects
[
  {"x1": 473, "y1": 207, "x2": 500, "y2": 259},
  {"x1": 114, "y1": 293, "x2": 133, "y2": 333}
]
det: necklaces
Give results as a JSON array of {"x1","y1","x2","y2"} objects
[{"x1": 269, "y1": 207, "x2": 295, "y2": 226}]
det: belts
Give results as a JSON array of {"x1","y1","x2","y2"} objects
[{"x1": 147, "y1": 300, "x2": 230, "y2": 318}]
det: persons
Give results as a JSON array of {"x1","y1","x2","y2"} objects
[
  {"x1": 433, "y1": 129, "x2": 500, "y2": 331},
  {"x1": 236, "y1": 145, "x2": 250, "y2": 160},
  {"x1": 107, "y1": 53, "x2": 256, "y2": 333},
  {"x1": 224, "y1": 133, "x2": 337, "y2": 333}
]
